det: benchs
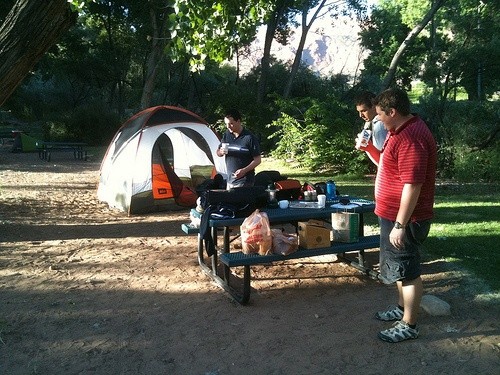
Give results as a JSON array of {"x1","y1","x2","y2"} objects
[
  {"x1": 181, "y1": 222, "x2": 382, "y2": 268},
  {"x1": 38, "y1": 147, "x2": 91, "y2": 161}
]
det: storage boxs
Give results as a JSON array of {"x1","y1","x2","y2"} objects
[{"x1": 298, "y1": 221, "x2": 332, "y2": 249}]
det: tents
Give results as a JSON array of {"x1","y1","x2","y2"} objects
[{"x1": 96, "y1": 105, "x2": 228, "y2": 217}]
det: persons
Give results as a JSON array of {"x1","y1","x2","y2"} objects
[
  {"x1": 355, "y1": 88, "x2": 437, "y2": 343},
  {"x1": 356, "y1": 91, "x2": 387, "y2": 151},
  {"x1": 217, "y1": 112, "x2": 262, "y2": 190}
]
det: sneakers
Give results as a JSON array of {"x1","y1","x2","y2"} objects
[
  {"x1": 376, "y1": 307, "x2": 404, "y2": 321},
  {"x1": 377, "y1": 320, "x2": 419, "y2": 343}
]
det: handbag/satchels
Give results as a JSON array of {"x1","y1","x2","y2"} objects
[
  {"x1": 240, "y1": 209, "x2": 273, "y2": 255},
  {"x1": 331, "y1": 203, "x2": 360, "y2": 243}
]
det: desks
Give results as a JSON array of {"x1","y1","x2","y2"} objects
[
  {"x1": 191, "y1": 196, "x2": 376, "y2": 305},
  {"x1": 38, "y1": 141, "x2": 88, "y2": 160}
]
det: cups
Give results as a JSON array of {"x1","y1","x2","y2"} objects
[
  {"x1": 311, "y1": 190, "x2": 317, "y2": 201},
  {"x1": 317, "y1": 195, "x2": 326, "y2": 208},
  {"x1": 304, "y1": 191, "x2": 312, "y2": 201},
  {"x1": 222, "y1": 142, "x2": 229, "y2": 154},
  {"x1": 279, "y1": 200, "x2": 288, "y2": 209}
]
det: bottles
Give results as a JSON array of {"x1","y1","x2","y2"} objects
[{"x1": 327, "y1": 180, "x2": 336, "y2": 200}]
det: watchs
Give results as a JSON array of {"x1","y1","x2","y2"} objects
[{"x1": 393, "y1": 221, "x2": 406, "y2": 229}]
